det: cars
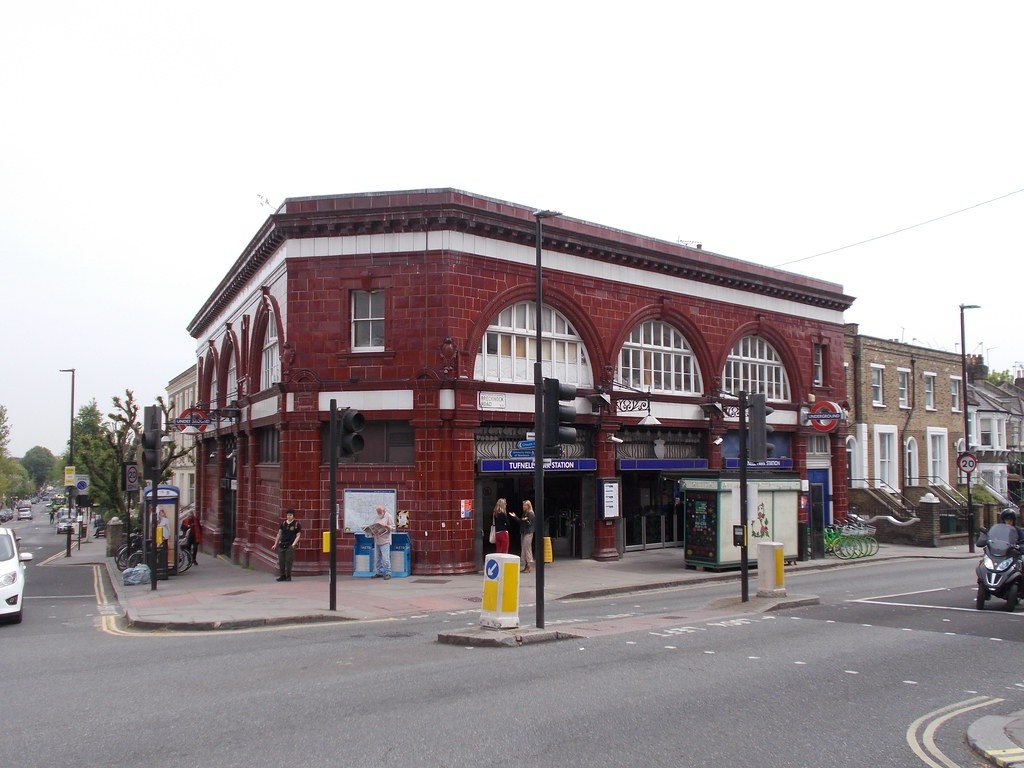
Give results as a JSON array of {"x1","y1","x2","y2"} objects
[
  {"x1": 0, "y1": 528, "x2": 33, "y2": 623},
  {"x1": 0, "y1": 488, "x2": 77, "y2": 535}
]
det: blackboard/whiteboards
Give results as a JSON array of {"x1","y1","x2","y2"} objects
[{"x1": 343, "y1": 489, "x2": 395, "y2": 533}]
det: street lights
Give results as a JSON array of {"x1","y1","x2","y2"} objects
[
  {"x1": 59, "y1": 369, "x2": 75, "y2": 557},
  {"x1": 959, "y1": 303, "x2": 982, "y2": 555},
  {"x1": 533, "y1": 207, "x2": 564, "y2": 630}
]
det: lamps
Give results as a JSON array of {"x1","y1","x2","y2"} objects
[
  {"x1": 220, "y1": 407, "x2": 240, "y2": 419},
  {"x1": 585, "y1": 377, "x2": 662, "y2": 425},
  {"x1": 698, "y1": 402, "x2": 723, "y2": 415}
]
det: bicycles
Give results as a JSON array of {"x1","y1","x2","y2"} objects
[
  {"x1": 807, "y1": 516, "x2": 879, "y2": 560},
  {"x1": 113, "y1": 529, "x2": 194, "y2": 575}
]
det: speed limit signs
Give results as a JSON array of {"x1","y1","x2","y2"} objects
[
  {"x1": 956, "y1": 453, "x2": 977, "y2": 473},
  {"x1": 125, "y1": 464, "x2": 139, "y2": 491}
]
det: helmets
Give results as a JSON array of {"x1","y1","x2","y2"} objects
[{"x1": 1001, "y1": 509, "x2": 1017, "y2": 520}]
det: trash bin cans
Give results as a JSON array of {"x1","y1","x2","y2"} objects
[
  {"x1": 797, "y1": 522, "x2": 809, "y2": 562},
  {"x1": 353, "y1": 532, "x2": 410, "y2": 577},
  {"x1": 145, "y1": 538, "x2": 168, "y2": 580}
]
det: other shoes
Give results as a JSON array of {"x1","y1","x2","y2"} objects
[
  {"x1": 371, "y1": 574, "x2": 383, "y2": 578},
  {"x1": 191, "y1": 560, "x2": 198, "y2": 565},
  {"x1": 384, "y1": 575, "x2": 391, "y2": 580},
  {"x1": 276, "y1": 576, "x2": 291, "y2": 581}
]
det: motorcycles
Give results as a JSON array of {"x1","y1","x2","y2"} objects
[{"x1": 975, "y1": 523, "x2": 1024, "y2": 613}]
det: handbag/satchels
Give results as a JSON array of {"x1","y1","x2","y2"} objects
[{"x1": 489, "y1": 525, "x2": 496, "y2": 543}]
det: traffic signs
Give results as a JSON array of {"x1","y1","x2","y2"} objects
[
  {"x1": 516, "y1": 440, "x2": 535, "y2": 449},
  {"x1": 507, "y1": 449, "x2": 535, "y2": 458}
]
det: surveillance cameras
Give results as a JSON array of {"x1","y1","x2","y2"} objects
[
  {"x1": 712, "y1": 437, "x2": 724, "y2": 445},
  {"x1": 609, "y1": 436, "x2": 623, "y2": 443}
]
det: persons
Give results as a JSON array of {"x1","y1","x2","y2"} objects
[
  {"x1": 49, "y1": 508, "x2": 55, "y2": 526},
  {"x1": 365, "y1": 505, "x2": 396, "y2": 580},
  {"x1": 93, "y1": 519, "x2": 106, "y2": 538},
  {"x1": 494, "y1": 498, "x2": 509, "y2": 553},
  {"x1": 180, "y1": 510, "x2": 202, "y2": 565},
  {"x1": 509, "y1": 500, "x2": 536, "y2": 573},
  {"x1": 1000, "y1": 508, "x2": 1024, "y2": 599},
  {"x1": 272, "y1": 509, "x2": 301, "y2": 582},
  {"x1": 675, "y1": 497, "x2": 684, "y2": 547},
  {"x1": 157, "y1": 510, "x2": 171, "y2": 571}
]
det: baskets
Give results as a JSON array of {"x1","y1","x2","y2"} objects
[{"x1": 180, "y1": 545, "x2": 186, "y2": 551}]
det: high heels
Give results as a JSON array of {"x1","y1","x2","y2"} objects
[{"x1": 520, "y1": 566, "x2": 531, "y2": 573}]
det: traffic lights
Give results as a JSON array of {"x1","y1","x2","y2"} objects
[
  {"x1": 141, "y1": 429, "x2": 162, "y2": 471},
  {"x1": 338, "y1": 408, "x2": 366, "y2": 457},
  {"x1": 748, "y1": 394, "x2": 774, "y2": 462},
  {"x1": 543, "y1": 377, "x2": 577, "y2": 448}
]
